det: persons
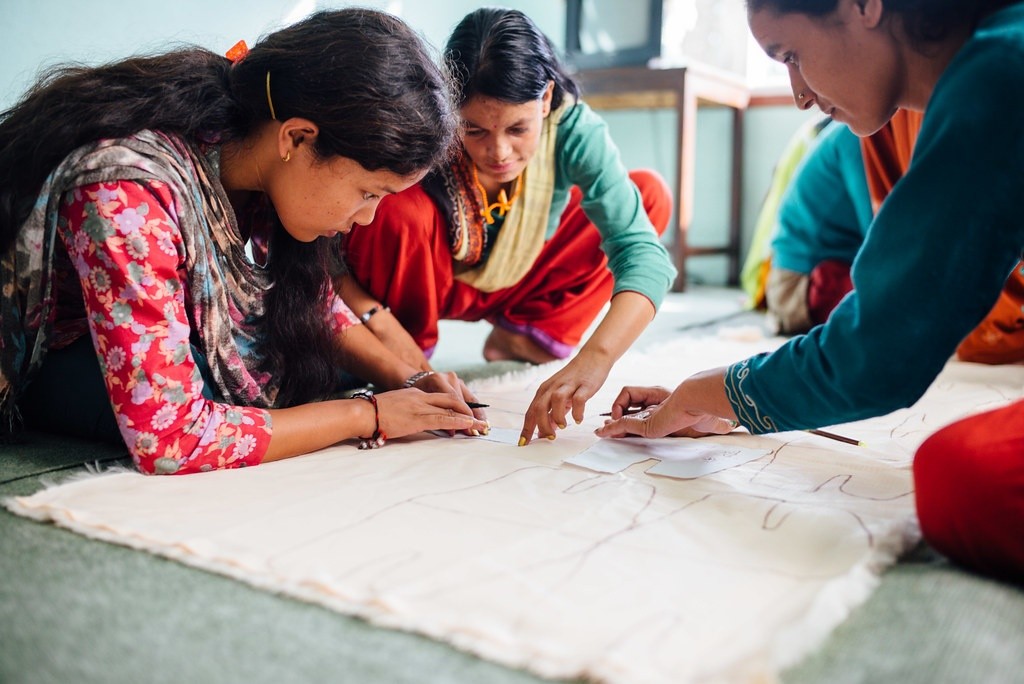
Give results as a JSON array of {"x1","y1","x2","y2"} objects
[
  {"x1": 316, "y1": 7, "x2": 679, "y2": 447},
  {"x1": 594, "y1": 1, "x2": 1024, "y2": 581},
  {"x1": 737, "y1": 103, "x2": 1024, "y2": 364},
  {"x1": 1, "y1": 9, "x2": 470, "y2": 478}
]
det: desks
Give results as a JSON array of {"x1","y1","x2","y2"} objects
[{"x1": 567, "y1": 66, "x2": 752, "y2": 294}]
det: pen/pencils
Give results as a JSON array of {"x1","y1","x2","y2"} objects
[
  {"x1": 598, "y1": 409, "x2": 642, "y2": 416},
  {"x1": 808, "y1": 429, "x2": 864, "y2": 447},
  {"x1": 466, "y1": 402, "x2": 490, "y2": 409}
]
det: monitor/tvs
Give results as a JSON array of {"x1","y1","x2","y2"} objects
[{"x1": 564, "y1": 1, "x2": 664, "y2": 76}]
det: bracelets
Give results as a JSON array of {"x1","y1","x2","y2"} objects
[
  {"x1": 400, "y1": 369, "x2": 434, "y2": 389},
  {"x1": 361, "y1": 303, "x2": 388, "y2": 323},
  {"x1": 351, "y1": 392, "x2": 386, "y2": 450}
]
